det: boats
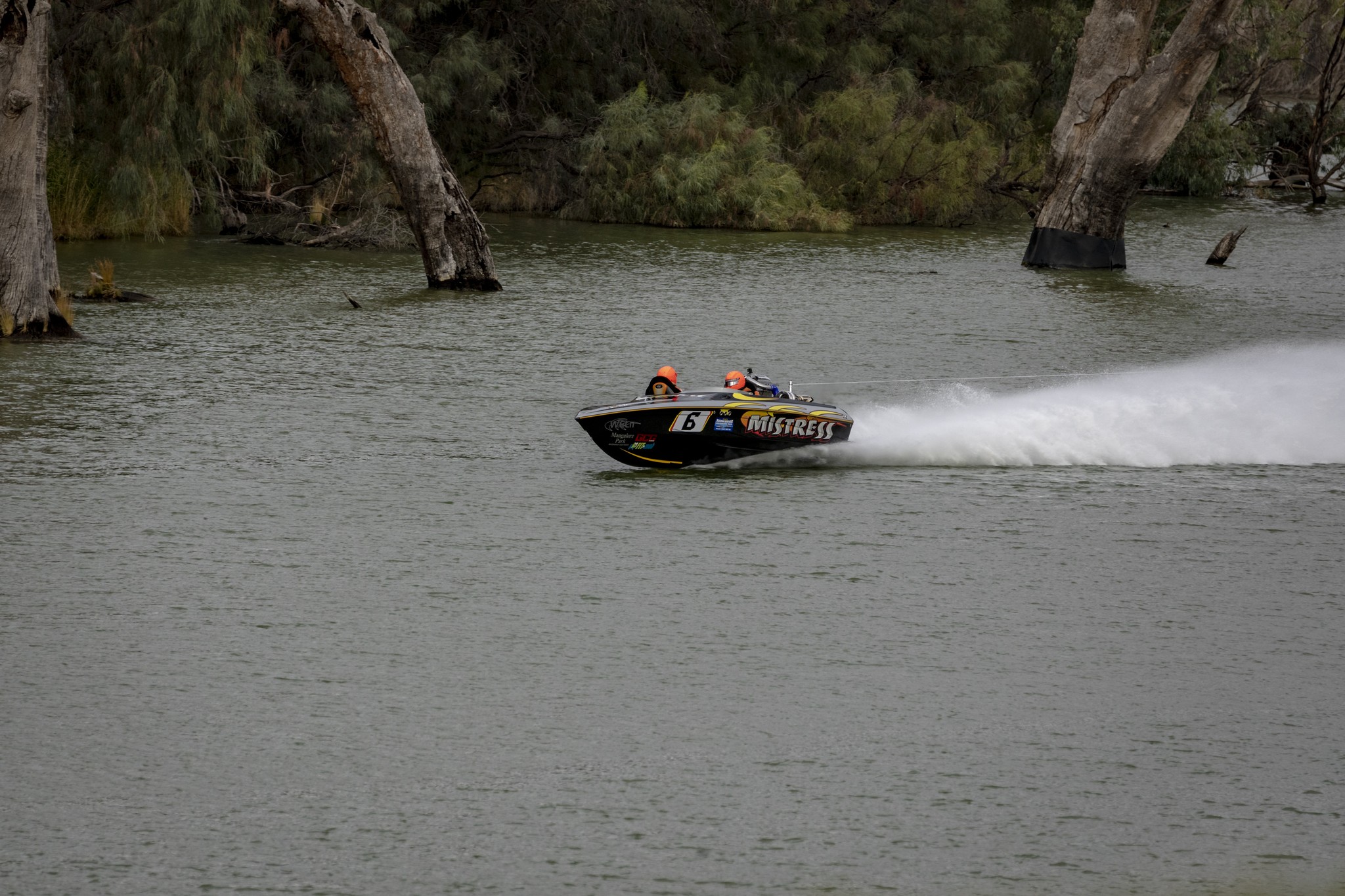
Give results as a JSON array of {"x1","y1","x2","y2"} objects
[{"x1": 573, "y1": 368, "x2": 854, "y2": 470}]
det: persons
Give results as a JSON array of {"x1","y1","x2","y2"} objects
[
  {"x1": 657, "y1": 366, "x2": 683, "y2": 392},
  {"x1": 724, "y1": 371, "x2": 753, "y2": 395}
]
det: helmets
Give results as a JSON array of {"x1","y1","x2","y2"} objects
[
  {"x1": 657, "y1": 366, "x2": 677, "y2": 386},
  {"x1": 725, "y1": 371, "x2": 745, "y2": 390}
]
